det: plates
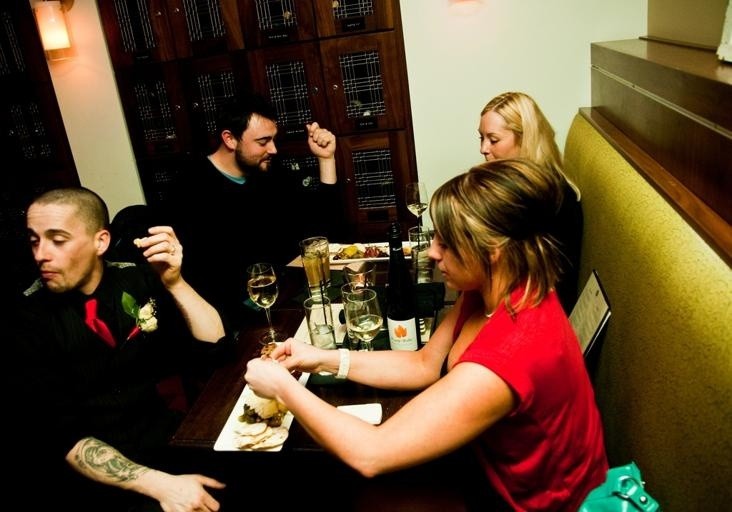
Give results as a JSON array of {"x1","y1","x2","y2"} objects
[
  {"x1": 328, "y1": 242, "x2": 391, "y2": 264},
  {"x1": 292, "y1": 303, "x2": 346, "y2": 347},
  {"x1": 336, "y1": 403, "x2": 382, "y2": 425},
  {"x1": 213, "y1": 369, "x2": 311, "y2": 453}
]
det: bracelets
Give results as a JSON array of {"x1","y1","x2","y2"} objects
[{"x1": 334, "y1": 348, "x2": 351, "y2": 382}]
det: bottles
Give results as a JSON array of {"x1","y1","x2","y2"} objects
[{"x1": 383, "y1": 221, "x2": 422, "y2": 352}]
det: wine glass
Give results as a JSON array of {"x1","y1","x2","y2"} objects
[
  {"x1": 404, "y1": 180, "x2": 430, "y2": 251},
  {"x1": 246, "y1": 263, "x2": 288, "y2": 345},
  {"x1": 340, "y1": 288, "x2": 384, "y2": 353}
]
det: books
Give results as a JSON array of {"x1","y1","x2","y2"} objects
[{"x1": 567, "y1": 270, "x2": 612, "y2": 358}]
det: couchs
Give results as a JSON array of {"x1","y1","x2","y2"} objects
[{"x1": 564, "y1": 107, "x2": 732, "y2": 512}]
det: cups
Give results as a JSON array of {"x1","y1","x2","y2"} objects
[
  {"x1": 302, "y1": 296, "x2": 337, "y2": 350},
  {"x1": 298, "y1": 237, "x2": 376, "y2": 302},
  {"x1": 408, "y1": 225, "x2": 431, "y2": 269}
]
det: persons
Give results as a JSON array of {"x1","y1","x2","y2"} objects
[
  {"x1": 1, "y1": 183, "x2": 230, "y2": 512},
  {"x1": 173, "y1": 93, "x2": 354, "y2": 328},
  {"x1": 478, "y1": 94, "x2": 579, "y2": 317},
  {"x1": 243, "y1": 158, "x2": 607, "y2": 510}
]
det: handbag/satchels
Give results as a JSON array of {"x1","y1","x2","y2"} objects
[{"x1": 578, "y1": 461, "x2": 662, "y2": 512}]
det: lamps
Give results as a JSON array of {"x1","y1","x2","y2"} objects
[{"x1": 34, "y1": 0, "x2": 75, "y2": 62}]
